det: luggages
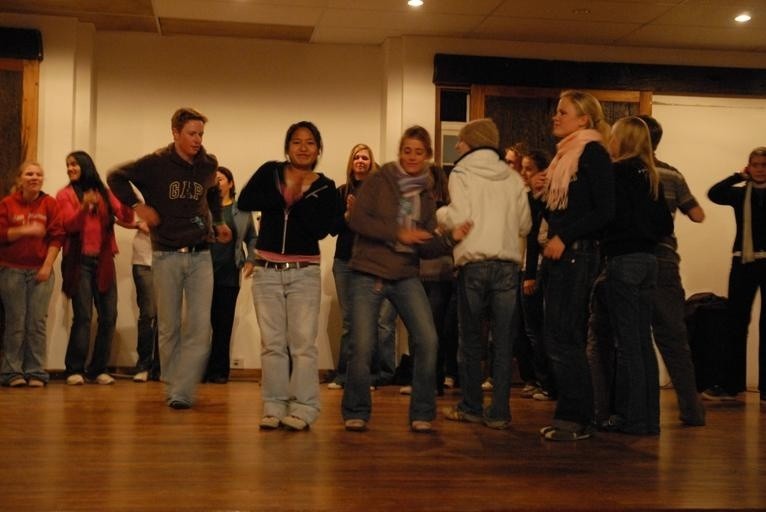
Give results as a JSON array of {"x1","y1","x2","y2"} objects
[{"x1": 685, "y1": 293, "x2": 729, "y2": 393}]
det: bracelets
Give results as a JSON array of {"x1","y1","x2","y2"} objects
[{"x1": 212, "y1": 221, "x2": 227, "y2": 226}]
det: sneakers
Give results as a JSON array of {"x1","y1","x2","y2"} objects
[
  {"x1": 280, "y1": 415, "x2": 307, "y2": 431},
  {"x1": 345, "y1": 419, "x2": 365, "y2": 430},
  {"x1": 7, "y1": 370, "x2": 229, "y2": 409},
  {"x1": 260, "y1": 416, "x2": 279, "y2": 427},
  {"x1": 411, "y1": 421, "x2": 430, "y2": 433}
]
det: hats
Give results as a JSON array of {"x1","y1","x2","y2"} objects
[{"x1": 459, "y1": 118, "x2": 500, "y2": 149}]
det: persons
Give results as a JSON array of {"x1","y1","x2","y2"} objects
[
  {"x1": 237, "y1": 121, "x2": 355, "y2": 431},
  {"x1": 702, "y1": 146, "x2": 765, "y2": 405},
  {"x1": 112, "y1": 216, "x2": 159, "y2": 383},
  {"x1": 201, "y1": 166, "x2": 258, "y2": 385},
  {"x1": 328, "y1": 89, "x2": 706, "y2": 443},
  {"x1": 107, "y1": 108, "x2": 232, "y2": 410},
  {"x1": 0, "y1": 159, "x2": 64, "y2": 387},
  {"x1": 55, "y1": 151, "x2": 134, "y2": 385}
]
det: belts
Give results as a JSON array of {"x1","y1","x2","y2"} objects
[
  {"x1": 254, "y1": 260, "x2": 320, "y2": 272},
  {"x1": 158, "y1": 244, "x2": 208, "y2": 253}
]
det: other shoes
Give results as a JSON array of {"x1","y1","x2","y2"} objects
[
  {"x1": 484, "y1": 416, "x2": 508, "y2": 430},
  {"x1": 541, "y1": 425, "x2": 588, "y2": 440},
  {"x1": 442, "y1": 406, "x2": 479, "y2": 423},
  {"x1": 328, "y1": 381, "x2": 341, "y2": 389},
  {"x1": 400, "y1": 384, "x2": 413, "y2": 394},
  {"x1": 678, "y1": 415, "x2": 706, "y2": 426},
  {"x1": 482, "y1": 382, "x2": 495, "y2": 391},
  {"x1": 600, "y1": 419, "x2": 655, "y2": 435},
  {"x1": 533, "y1": 391, "x2": 550, "y2": 401},
  {"x1": 520, "y1": 385, "x2": 537, "y2": 398},
  {"x1": 443, "y1": 376, "x2": 455, "y2": 388}
]
